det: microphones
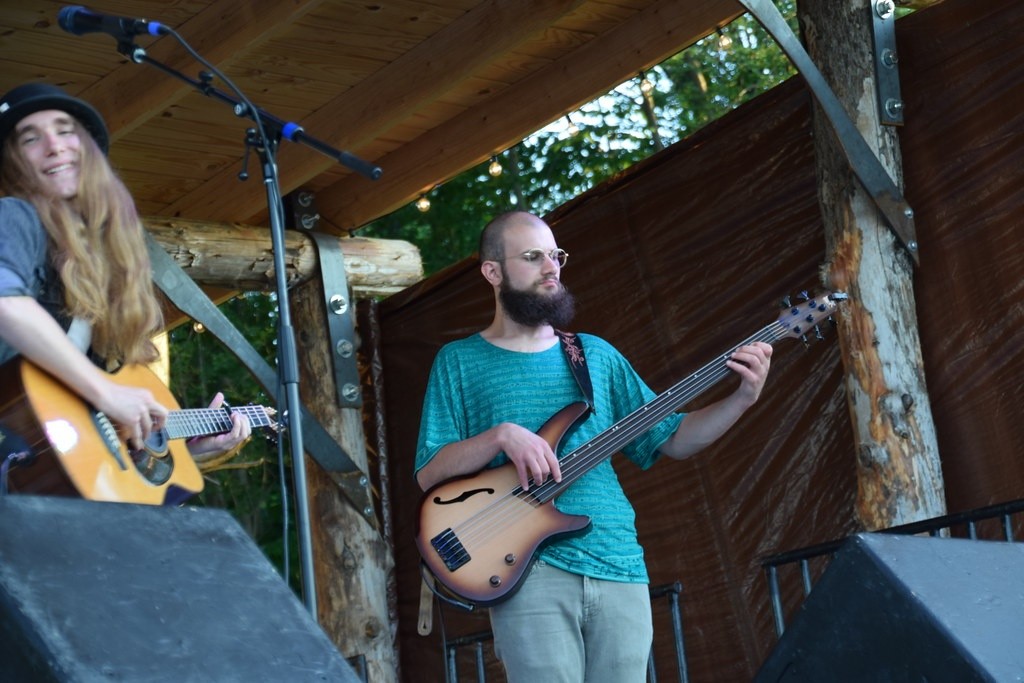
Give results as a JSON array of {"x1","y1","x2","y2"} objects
[{"x1": 57, "y1": 6, "x2": 171, "y2": 37}]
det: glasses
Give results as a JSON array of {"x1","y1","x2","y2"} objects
[{"x1": 492, "y1": 248, "x2": 568, "y2": 268}]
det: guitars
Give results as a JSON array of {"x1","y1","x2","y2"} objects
[
  {"x1": 412, "y1": 286, "x2": 848, "y2": 606},
  {"x1": 0, "y1": 354, "x2": 289, "y2": 504}
]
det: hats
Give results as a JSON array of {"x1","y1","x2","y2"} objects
[{"x1": 0, "y1": 81, "x2": 109, "y2": 156}]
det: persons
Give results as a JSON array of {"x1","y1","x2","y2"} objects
[
  {"x1": 0, "y1": 86, "x2": 251, "y2": 506},
  {"x1": 414, "y1": 211, "x2": 773, "y2": 683}
]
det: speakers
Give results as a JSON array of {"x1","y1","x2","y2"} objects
[
  {"x1": 0, "y1": 493, "x2": 366, "y2": 683},
  {"x1": 752, "y1": 532, "x2": 1024, "y2": 683}
]
